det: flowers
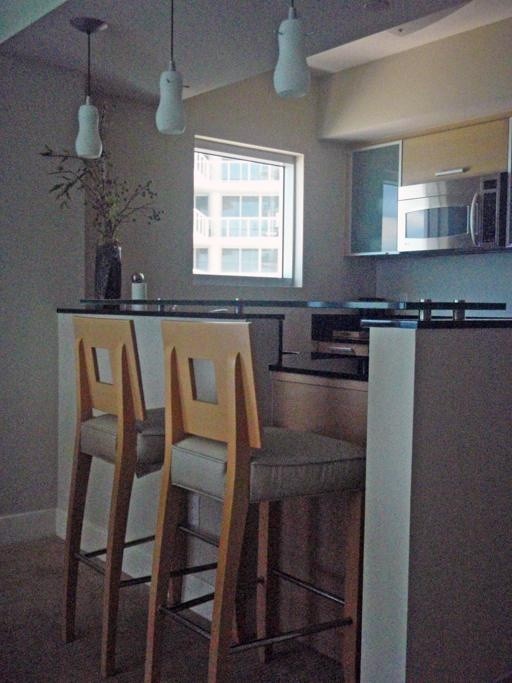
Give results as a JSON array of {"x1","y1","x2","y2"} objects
[{"x1": 40, "y1": 104, "x2": 163, "y2": 309}]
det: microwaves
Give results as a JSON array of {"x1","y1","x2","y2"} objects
[{"x1": 396, "y1": 170, "x2": 508, "y2": 256}]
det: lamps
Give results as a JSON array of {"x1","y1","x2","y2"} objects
[
  {"x1": 69, "y1": 17, "x2": 108, "y2": 160},
  {"x1": 272, "y1": 0, "x2": 313, "y2": 97},
  {"x1": 153, "y1": 0, "x2": 187, "y2": 136}
]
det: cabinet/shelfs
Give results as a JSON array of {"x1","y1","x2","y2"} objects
[{"x1": 401, "y1": 115, "x2": 508, "y2": 185}]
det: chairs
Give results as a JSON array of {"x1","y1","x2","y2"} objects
[
  {"x1": 59, "y1": 315, "x2": 242, "y2": 673},
  {"x1": 144, "y1": 316, "x2": 363, "y2": 683}
]
